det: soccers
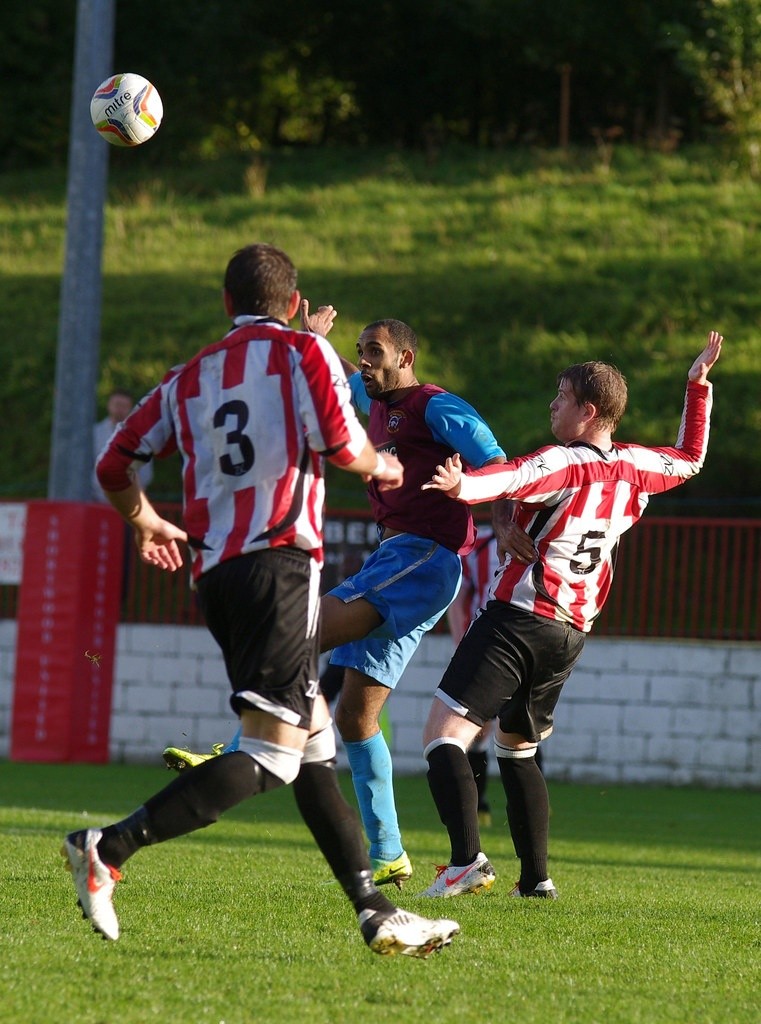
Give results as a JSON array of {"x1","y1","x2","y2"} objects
[{"x1": 90, "y1": 73, "x2": 163, "y2": 147}]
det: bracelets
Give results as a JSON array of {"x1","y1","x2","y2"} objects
[{"x1": 372, "y1": 453, "x2": 386, "y2": 478}]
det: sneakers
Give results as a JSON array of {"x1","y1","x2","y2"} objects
[
  {"x1": 58, "y1": 827, "x2": 122, "y2": 943},
  {"x1": 162, "y1": 740, "x2": 220, "y2": 775},
  {"x1": 367, "y1": 848, "x2": 418, "y2": 893},
  {"x1": 354, "y1": 896, "x2": 461, "y2": 962},
  {"x1": 422, "y1": 852, "x2": 496, "y2": 901},
  {"x1": 505, "y1": 877, "x2": 557, "y2": 907}
]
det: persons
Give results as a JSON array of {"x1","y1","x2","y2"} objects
[
  {"x1": 161, "y1": 298, "x2": 540, "y2": 887},
  {"x1": 58, "y1": 244, "x2": 462, "y2": 960},
  {"x1": 93, "y1": 389, "x2": 155, "y2": 621},
  {"x1": 422, "y1": 331, "x2": 726, "y2": 898}
]
